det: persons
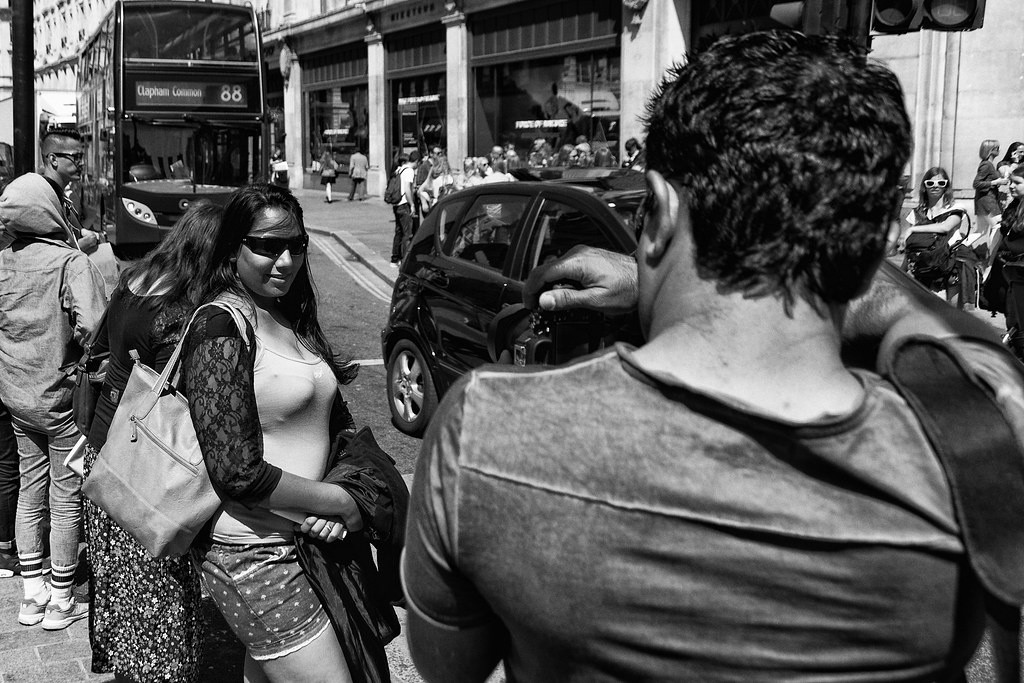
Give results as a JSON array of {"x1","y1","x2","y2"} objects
[
  {"x1": 882, "y1": 139, "x2": 1024, "y2": 362},
  {"x1": 319, "y1": 155, "x2": 339, "y2": 204},
  {"x1": 79, "y1": 199, "x2": 250, "y2": 682},
  {"x1": 398, "y1": 32, "x2": 1024, "y2": 683},
  {"x1": 0, "y1": 172, "x2": 110, "y2": 632},
  {"x1": 346, "y1": 147, "x2": 369, "y2": 201},
  {"x1": 390, "y1": 134, "x2": 645, "y2": 267},
  {"x1": 1, "y1": 128, "x2": 107, "y2": 579},
  {"x1": 271, "y1": 146, "x2": 283, "y2": 186},
  {"x1": 174, "y1": 183, "x2": 365, "y2": 682}
]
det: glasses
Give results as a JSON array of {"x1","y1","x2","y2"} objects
[
  {"x1": 924, "y1": 179, "x2": 948, "y2": 189},
  {"x1": 634, "y1": 172, "x2": 693, "y2": 244},
  {"x1": 241, "y1": 232, "x2": 309, "y2": 257},
  {"x1": 46, "y1": 153, "x2": 83, "y2": 165}
]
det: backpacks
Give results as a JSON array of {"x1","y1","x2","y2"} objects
[
  {"x1": 384, "y1": 166, "x2": 411, "y2": 204},
  {"x1": 905, "y1": 209, "x2": 971, "y2": 281}
]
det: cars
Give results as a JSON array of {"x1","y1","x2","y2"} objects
[{"x1": 379, "y1": 157, "x2": 672, "y2": 439}]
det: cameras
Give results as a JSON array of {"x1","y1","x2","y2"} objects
[{"x1": 487, "y1": 283, "x2": 613, "y2": 367}]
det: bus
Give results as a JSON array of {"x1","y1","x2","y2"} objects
[{"x1": 73, "y1": 0, "x2": 287, "y2": 246}]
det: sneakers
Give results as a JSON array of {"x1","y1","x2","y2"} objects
[
  {"x1": 19, "y1": 582, "x2": 51, "y2": 626},
  {"x1": 0, "y1": 553, "x2": 52, "y2": 578},
  {"x1": 42, "y1": 590, "x2": 90, "y2": 630}
]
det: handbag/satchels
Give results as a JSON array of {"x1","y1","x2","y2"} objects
[
  {"x1": 978, "y1": 255, "x2": 1006, "y2": 313},
  {"x1": 73, "y1": 352, "x2": 110, "y2": 438},
  {"x1": 80, "y1": 302, "x2": 250, "y2": 561}
]
